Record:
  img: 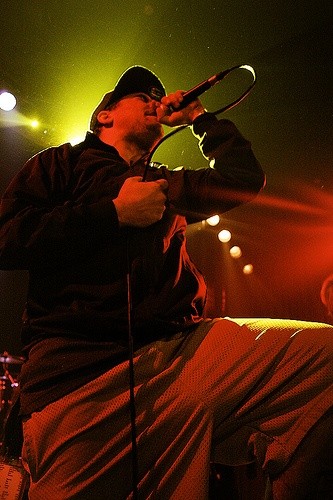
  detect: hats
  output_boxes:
[88,65,167,136]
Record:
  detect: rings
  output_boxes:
[156,180,163,189]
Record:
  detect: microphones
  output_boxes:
[159,65,235,116]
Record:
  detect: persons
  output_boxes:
[2,64,333,500]
